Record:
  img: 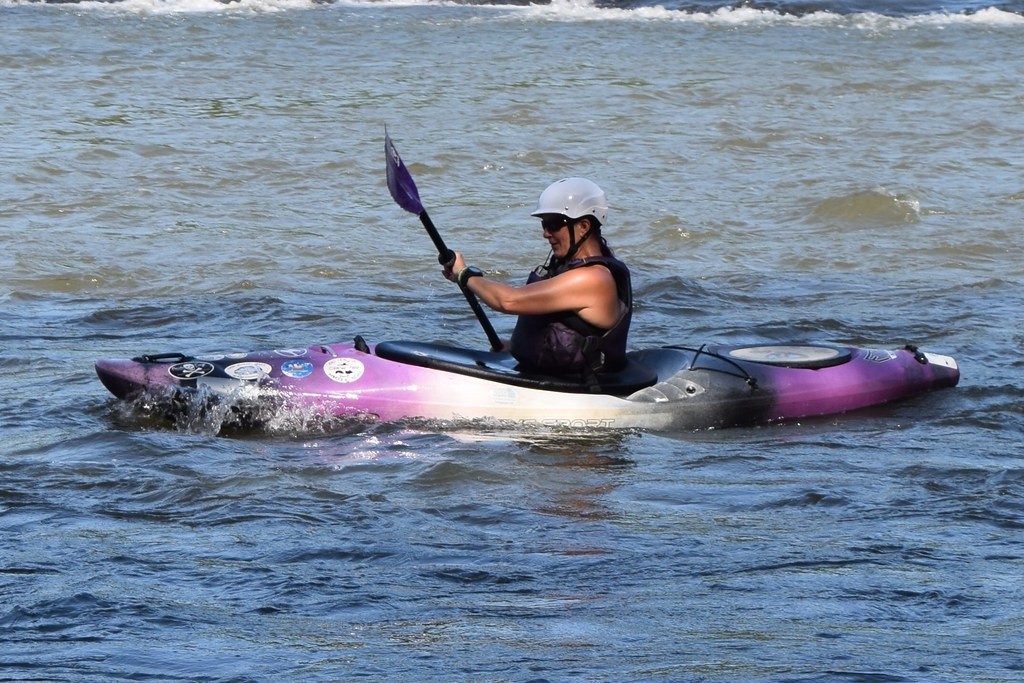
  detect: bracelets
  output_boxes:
[458,266,468,282]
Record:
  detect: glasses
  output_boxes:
[541,218,578,231]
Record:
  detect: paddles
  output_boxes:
[384,125,502,353]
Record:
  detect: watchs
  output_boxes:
[461,266,483,286]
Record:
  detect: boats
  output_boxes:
[95,338,963,440]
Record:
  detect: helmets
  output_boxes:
[531,178,608,225]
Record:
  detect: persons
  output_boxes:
[441,178,631,375]
